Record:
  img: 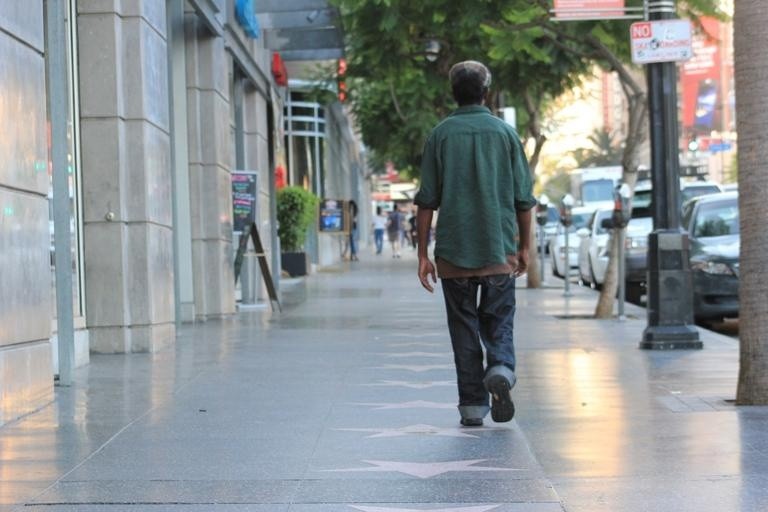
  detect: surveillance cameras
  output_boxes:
[306,9,320,23]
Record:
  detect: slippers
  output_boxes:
[491,375,515,423]
[460,416,484,426]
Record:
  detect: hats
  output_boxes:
[449,60,492,93]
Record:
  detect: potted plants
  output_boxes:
[274,184,321,277]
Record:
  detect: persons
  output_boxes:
[372,203,433,258]
[412,59,538,427]
[349,199,359,262]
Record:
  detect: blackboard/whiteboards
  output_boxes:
[231,170,258,234]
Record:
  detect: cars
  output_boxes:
[537,164,740,330]
